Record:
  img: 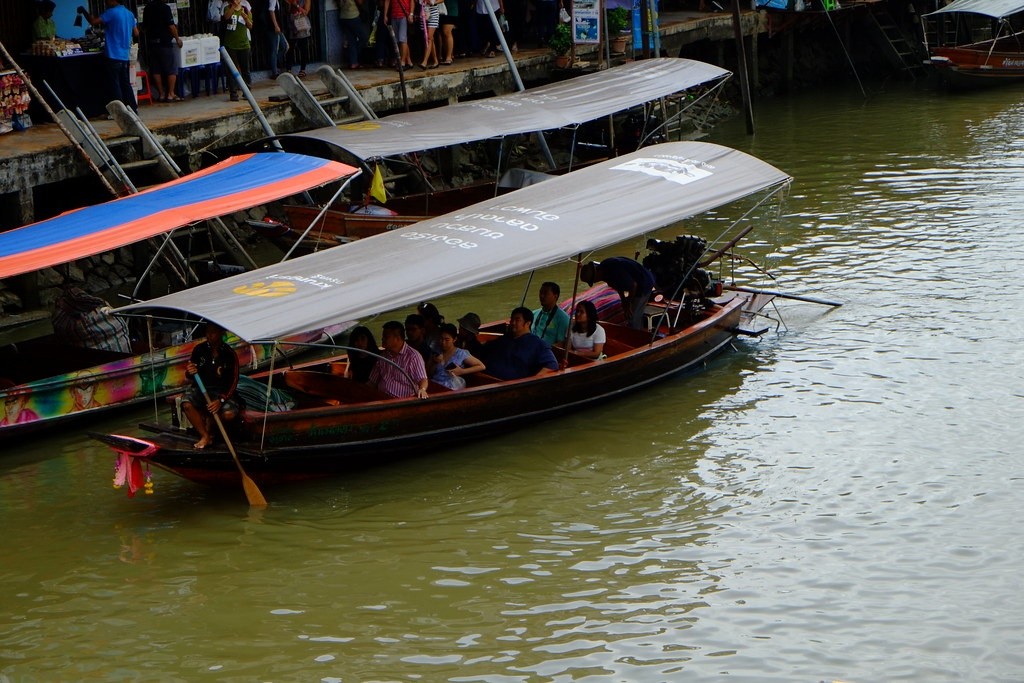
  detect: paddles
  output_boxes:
[720,284,846,306]
[186,357,267,505]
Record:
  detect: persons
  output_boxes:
[418,0,459,69]
[335,0,383,69]
[33,0,55,42]
[405,302,486,390]
[482,307,559,380]
[457,313,486,361]
[471,0,505,58]
[77,0,139,117]
[219,0,253,101]
[181,321,240,449]
[52,289,133,352]
[530,282,569,344]
[368,321,430,399]
[580,256,652,328]
[566,301,606,359]
[383,0,415,71]
[143,0,184,101]
[348,171,394,215]
[344,326,380,381]
[267,0,311,79]
[532,0,564,47]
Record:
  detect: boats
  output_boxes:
[245,57,734,260]
[0,152,361,430]
[920,0,1024,88]
[85,142,795,491]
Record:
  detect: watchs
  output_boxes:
[219,398,225,405]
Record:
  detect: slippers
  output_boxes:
[158,96,167,103]
[167,95,185,102]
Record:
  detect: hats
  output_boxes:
[457,313,481,335]
[581,261,596,288]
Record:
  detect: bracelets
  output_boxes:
[410,13,414,16]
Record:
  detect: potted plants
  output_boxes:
[610,6,628,54]
[548,25,572,69]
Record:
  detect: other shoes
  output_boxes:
[242,88,251,97]
[396,44,518,72]
[298,71,306,78]
[229,94,240,102]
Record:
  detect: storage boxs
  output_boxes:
[172,37,201,70]
[197,36,221,64]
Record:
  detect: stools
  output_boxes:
[176,62,227,100]
[152,324,193,345]
[643,306,670,332]
[136,72,153,108]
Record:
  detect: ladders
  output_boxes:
[56,100,242,287]
[276,65,432,199]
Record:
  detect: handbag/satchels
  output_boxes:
[293,10,312,34]
